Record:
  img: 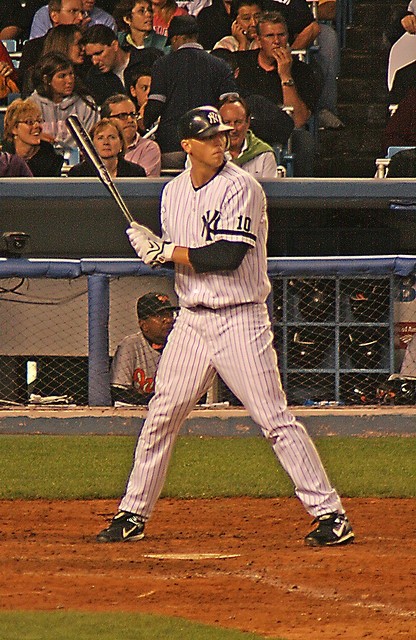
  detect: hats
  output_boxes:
[136,292,181,318]
[163,13,200,48]
[177,105,235,141]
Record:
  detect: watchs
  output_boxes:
[281,77,297,88]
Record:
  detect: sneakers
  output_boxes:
[303,511,356,547]
[316,108,346,130]
[96,511,146,543]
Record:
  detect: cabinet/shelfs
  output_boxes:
[267,276,395,405]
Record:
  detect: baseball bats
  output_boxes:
[65,114,163,271]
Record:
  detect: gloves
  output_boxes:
[138,239,177,267]
[125,220,161,251]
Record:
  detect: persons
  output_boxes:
[196,0,232,50]
[0,40,19,107]
[0,141,35,177]
[78,23,165,106]
[137,14,245,177]
[209,48,295,146]
[183,90,278,179]
[121,0,190,37]
[123,62,154,117]
[1,95,64,176]
[108,290,206,406]
[68,119,146,177]
[93,104,356,549]
[384,2,416,106]
[262,1,347,132]
[210,0,266,52]
[16,0,92,93]
[28,0,119,41]
[99,93,162,176]
[111,0,173,56]
[232,11,319,176]
[20,23,101,100]
[23,49,101,148]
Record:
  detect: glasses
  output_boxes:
[16,119,45,126]
[58,7,89,20]
[108,112,140,121]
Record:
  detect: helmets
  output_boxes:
[348,281,388,322]
[346,334,385,369]
[345,377,376,406]
[379,376,416,405]
[296,281,336,320]
[289,330,331,368]
